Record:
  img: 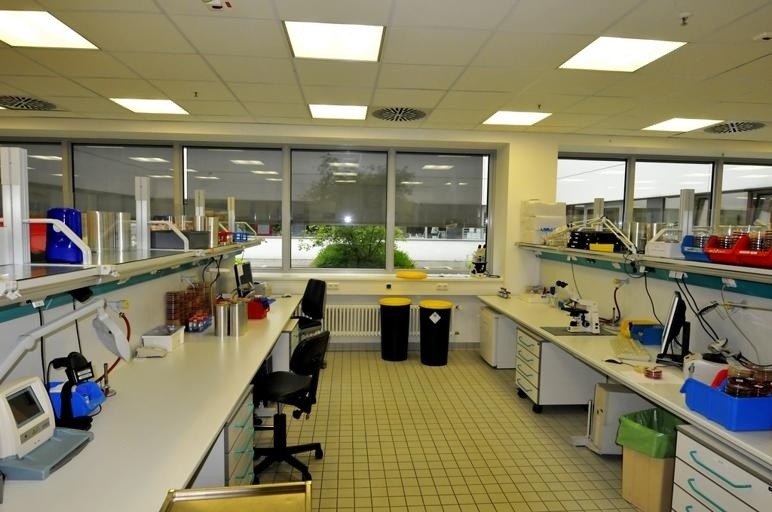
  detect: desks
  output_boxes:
[0,295,304,512]
[477,294,772,511]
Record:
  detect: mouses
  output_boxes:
[281,293,291,298]
[603,357,622,363]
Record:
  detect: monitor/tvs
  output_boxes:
[234,262,255,299]
[660,291,690,360]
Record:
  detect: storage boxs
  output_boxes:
[670,424,771,511]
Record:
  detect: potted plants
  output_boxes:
[470,244,489,274]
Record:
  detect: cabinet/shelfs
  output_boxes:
[515,324,607,414]
[224,385,254,486]
[478,306,516,370]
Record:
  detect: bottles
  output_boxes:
[718,225,771,252]
[663,223,681,244]
[691,226,711,247]
[726,364,771,398]
[189,311,209,332]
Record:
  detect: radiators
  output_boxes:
[291,304,455,337]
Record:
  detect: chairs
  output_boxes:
[289,278,326,341]
[251,330,330,485]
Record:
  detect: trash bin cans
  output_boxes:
[620,407,691,512]
[379,298,452,365]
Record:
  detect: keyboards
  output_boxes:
[608,336,652,361]
